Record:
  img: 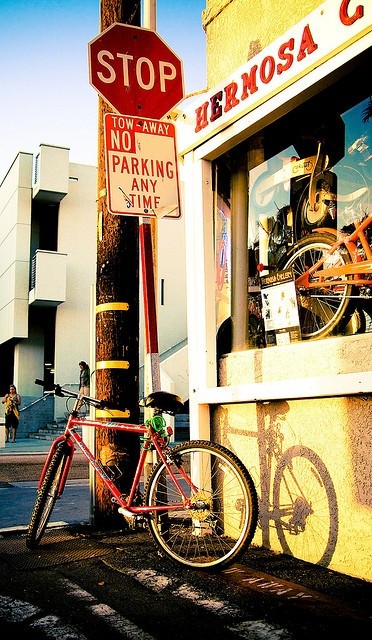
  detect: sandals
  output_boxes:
[5,440,9,443]
[11,440,16,443]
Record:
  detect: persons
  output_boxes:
[75,360,90,412]
[3,383,21,442]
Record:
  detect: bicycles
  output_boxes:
[251,139,334,228]
[24,376,257,572]
[210,397,340,571]
[274,184,372,340]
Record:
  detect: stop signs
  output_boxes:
[86,21,186,120]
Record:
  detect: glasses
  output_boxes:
[10,388,14,390]
